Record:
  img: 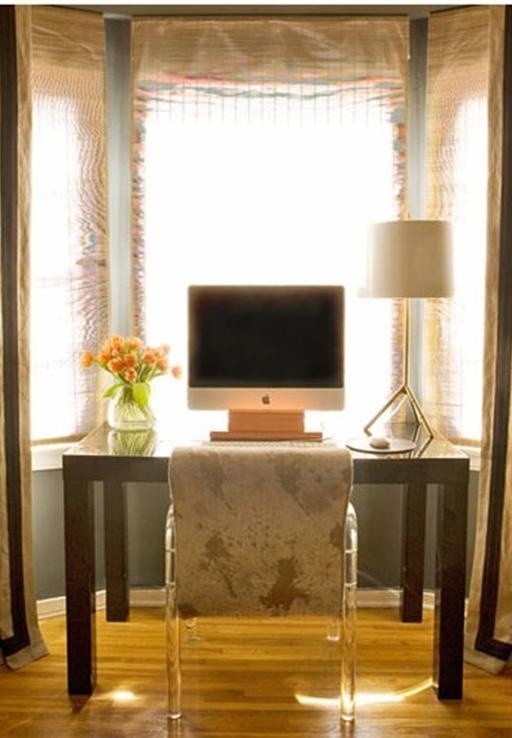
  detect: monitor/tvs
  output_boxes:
[187,284,345,442]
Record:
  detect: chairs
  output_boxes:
[153,444,362,736]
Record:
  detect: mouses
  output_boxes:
[368,434,391,449]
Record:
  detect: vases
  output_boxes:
[107,388,154,437]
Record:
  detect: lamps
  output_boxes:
[361,217,456,456]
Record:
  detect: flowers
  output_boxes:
[80,332,177,429]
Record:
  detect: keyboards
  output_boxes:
[203,441,338,447]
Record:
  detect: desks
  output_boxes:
[60,414,476,692]
